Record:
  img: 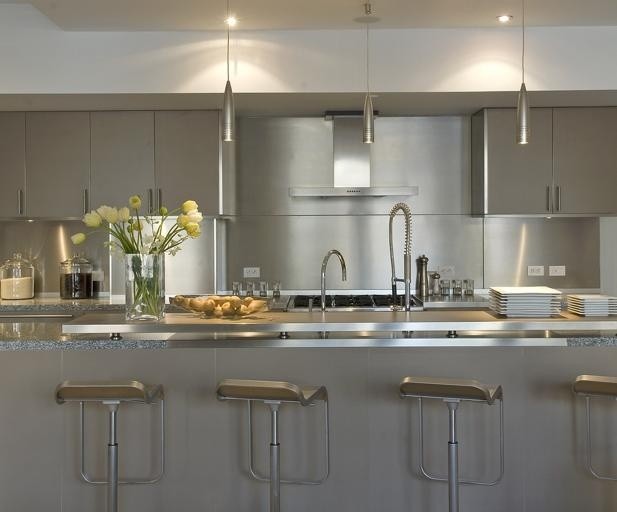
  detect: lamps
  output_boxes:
[516,0,530,145]
[222,0,235,142]
[363,4,374,143]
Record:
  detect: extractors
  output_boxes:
[287,110,419,199]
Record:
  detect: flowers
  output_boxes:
[70,194,205,315]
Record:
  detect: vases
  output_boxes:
[124,253,166,320]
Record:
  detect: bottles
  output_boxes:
[58,251,94,300]
[0,252,36,301]
[417,255,430,297]
[432,270,441,295]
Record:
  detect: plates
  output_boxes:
[486,284,617,319]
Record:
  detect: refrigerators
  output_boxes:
[108,215,227,301]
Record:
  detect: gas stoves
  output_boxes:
[287,291,425,313]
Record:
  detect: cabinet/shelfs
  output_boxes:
[90,109,223,217]
[0,111,91,222]
[470,104,617,220]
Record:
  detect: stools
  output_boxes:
[216,378,330,512]
[398,376,504,512]
[55,380,164,512]
[570,374,617,482]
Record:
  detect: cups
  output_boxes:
[259,280,268,298]
[245,280,255,296]
[464,279,474,296]
[453,279,462,296]
[232,281,242,296]
[271,279,282,298]
[441,280,450,296]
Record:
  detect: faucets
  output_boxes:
[388,203,411,313]
[320,250,347,311]
